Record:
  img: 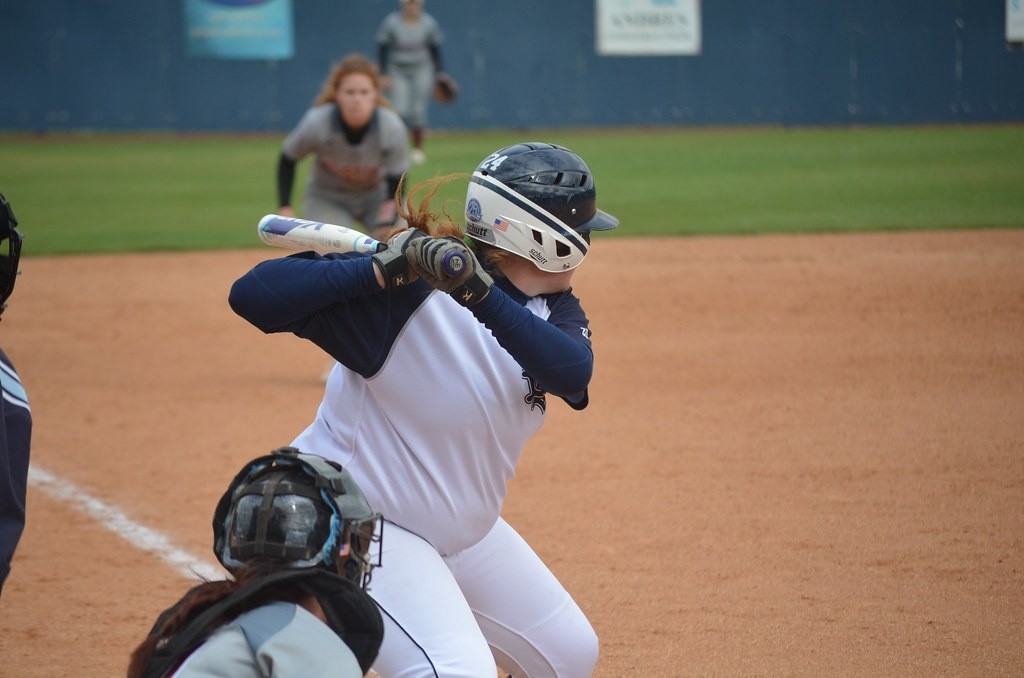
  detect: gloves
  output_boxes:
[407,235,494,308]
[372,226,432,293]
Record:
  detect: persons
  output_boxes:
[127,446,385,678]
[229,141,622,678]
[374,0,457,163]
[0,192,34,598]
[274,53,424,243]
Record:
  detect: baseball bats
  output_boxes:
[256,212,468,279]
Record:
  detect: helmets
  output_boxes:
[212,447,376,576]
[464,141,619,274]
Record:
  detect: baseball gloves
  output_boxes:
[435,72,461,104]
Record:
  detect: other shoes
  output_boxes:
[409,148,423,163]
